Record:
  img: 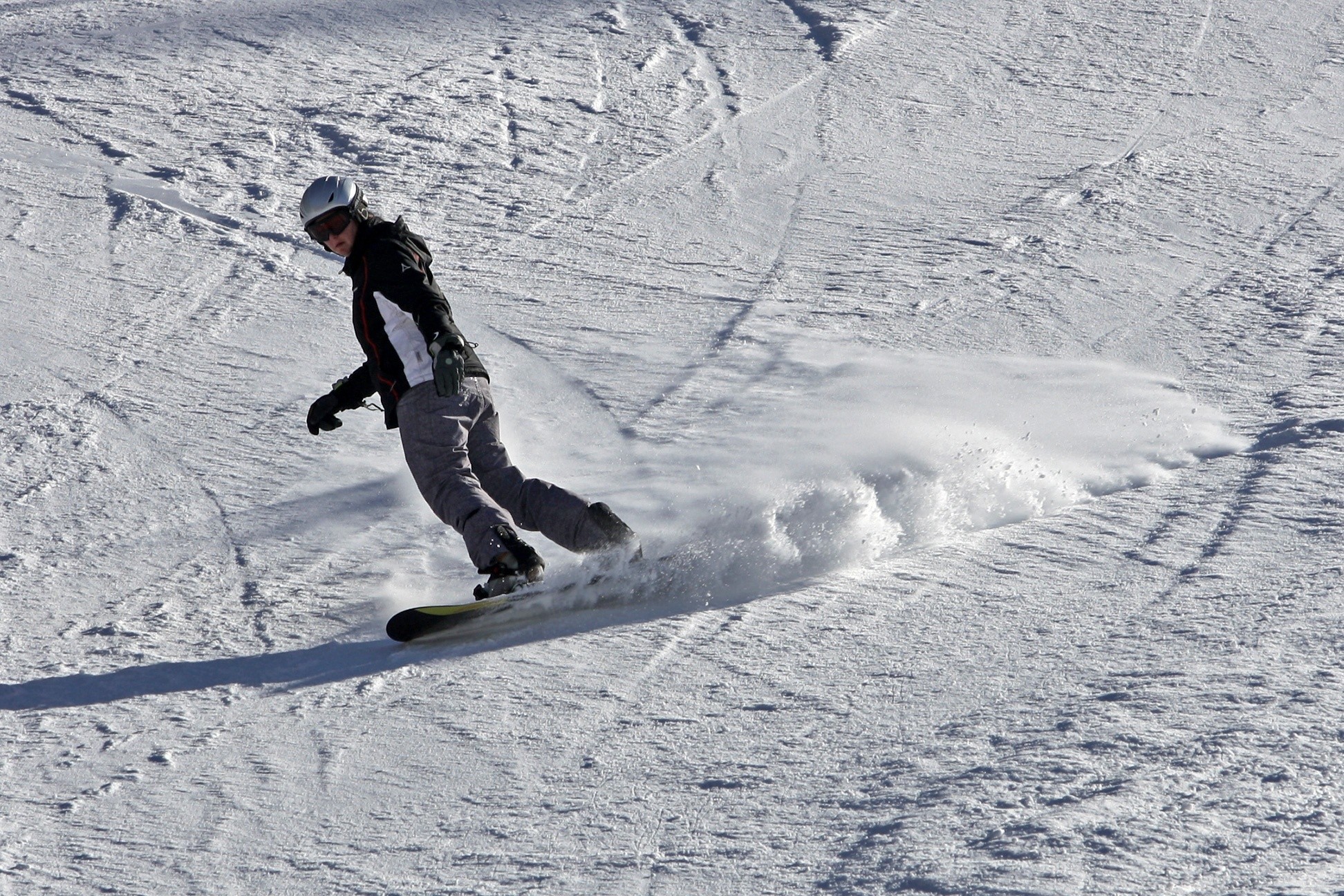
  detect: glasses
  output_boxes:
[306,207,351,242]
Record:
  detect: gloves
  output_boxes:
[429,335,465,397]
[307,390,343,435]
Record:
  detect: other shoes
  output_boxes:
[472,560,547,600]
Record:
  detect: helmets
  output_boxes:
[299,175,368,232]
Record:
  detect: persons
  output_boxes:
[300,175,644,605]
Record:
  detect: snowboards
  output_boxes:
[384,554,681,644]
